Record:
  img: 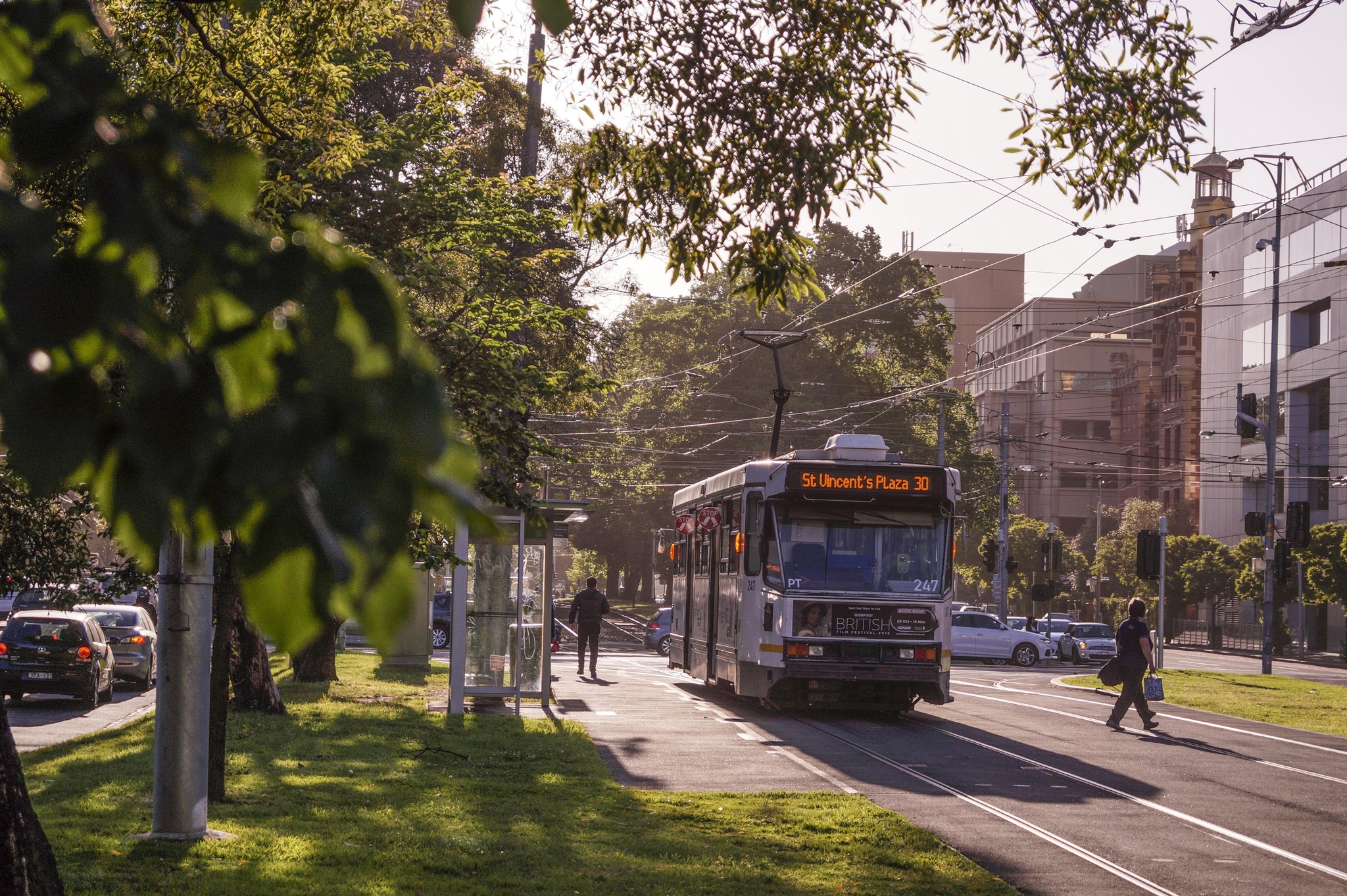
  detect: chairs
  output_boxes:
[785,543,825,580]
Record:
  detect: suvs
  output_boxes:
[950,611,1052,668]
[432,592,562,650]
[1057,622,1117,665]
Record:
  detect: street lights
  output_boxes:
[1226,153,1285,676]
[1199,430,1305,662]
[1019,463,1102,622]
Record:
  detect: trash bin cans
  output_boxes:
[1210,625,1223,649]
[508,623,542,692]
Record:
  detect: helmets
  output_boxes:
[137,586,150,600]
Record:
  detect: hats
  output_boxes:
[800,602,828,617]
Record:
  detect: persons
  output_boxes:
[568,576,610,677]
[555,586,566,599]
[551,603,555,638]
[1106,599,1159,731]
[1026,616,1039,634]
[797,600,828,638]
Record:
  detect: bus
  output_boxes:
[658,328,970,716]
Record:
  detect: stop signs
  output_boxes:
[732,506,740,530]
[675,514,696,535]
[699,506,722,530]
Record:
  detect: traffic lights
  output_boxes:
[1050,580,1062,598]
[1006,556,1019,574]
[553,573,557,578]
[1031,583,1049,602]
[982,538,995,573]
[1274,545,1291,579]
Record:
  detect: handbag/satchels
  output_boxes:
[550,638,560,653]
[1097,656,1122,686]
[1144,671,1164,701]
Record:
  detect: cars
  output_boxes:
[0,609,121,709]
[951,601,1074,655]
[644,607,671,657]
[54,603,158,690]
[0,568,160,636]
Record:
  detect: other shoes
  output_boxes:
[1143,721,1159,730]
[576,670,584,675]
[591,672,597,677]
[1106,718,1125,731]
